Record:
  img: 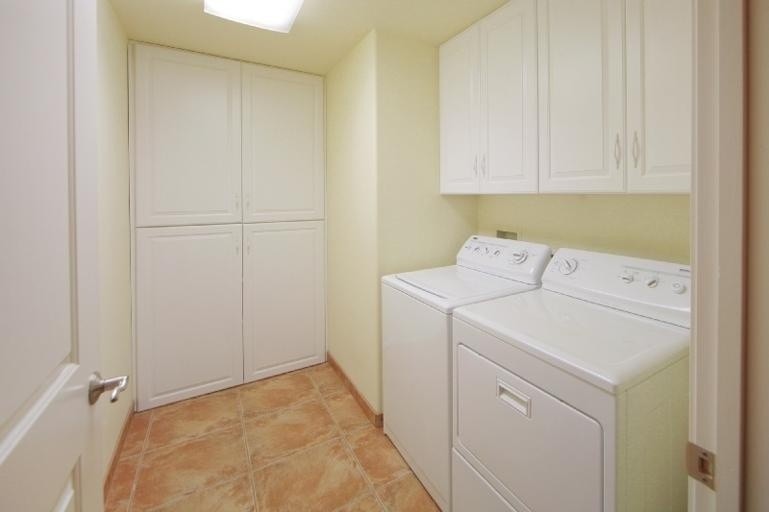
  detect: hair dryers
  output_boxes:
[451,248,692,512]
[381,235,552,512]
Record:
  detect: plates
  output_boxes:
[437,1,538,198]
[538,0,695,197]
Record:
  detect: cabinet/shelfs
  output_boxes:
[538,0,695,197]
[130,221,329,411]
[437,1,538,198]
[126,42,331,227]
